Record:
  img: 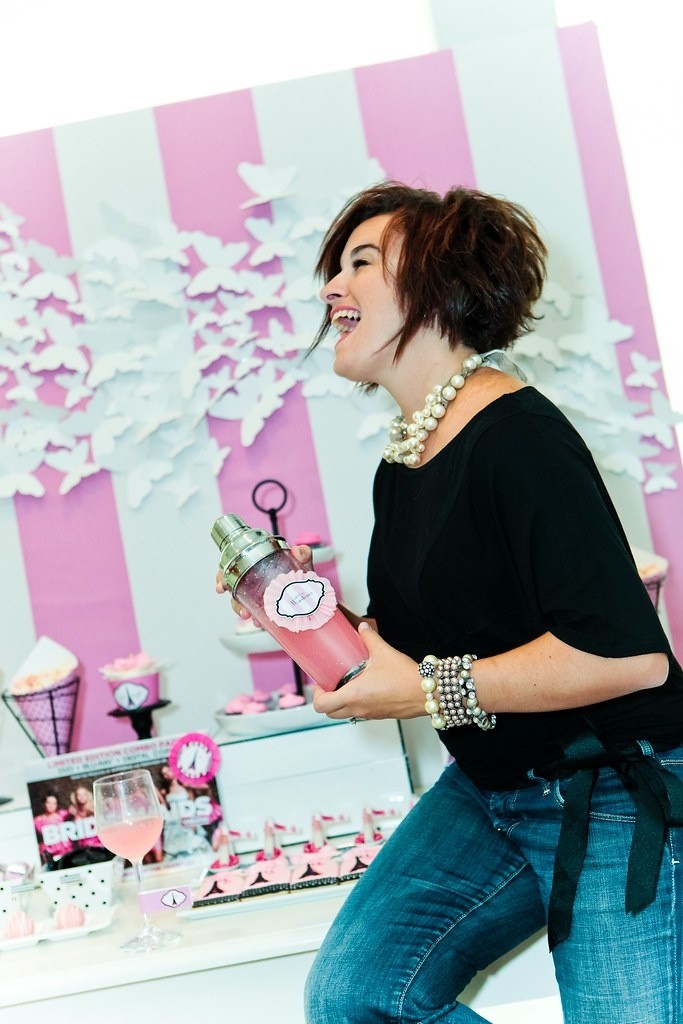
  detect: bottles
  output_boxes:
[210,514,371,694]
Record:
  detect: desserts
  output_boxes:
[295,532,321,548]
[224,681,305,714]
[235,616,262,634]
[197,832,384,905]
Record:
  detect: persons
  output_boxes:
[35,764,222,871]
[214,179,683,1024]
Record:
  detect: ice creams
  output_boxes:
[97,652,158,676]
[9,635,81,759]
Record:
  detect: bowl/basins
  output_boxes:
[0,857,129,911]
[104,668,160,710]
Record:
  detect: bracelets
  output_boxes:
[417,653,497,732]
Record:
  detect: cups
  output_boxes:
[134,884,192,915]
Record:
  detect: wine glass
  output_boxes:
[92,769,184,955]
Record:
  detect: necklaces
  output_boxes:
[382,349,528,468]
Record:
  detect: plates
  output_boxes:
[214,685,324,736]
[0,905,119,951]
[219,630,285,654]
[179,858,362,923]
[203,793,419,864]
